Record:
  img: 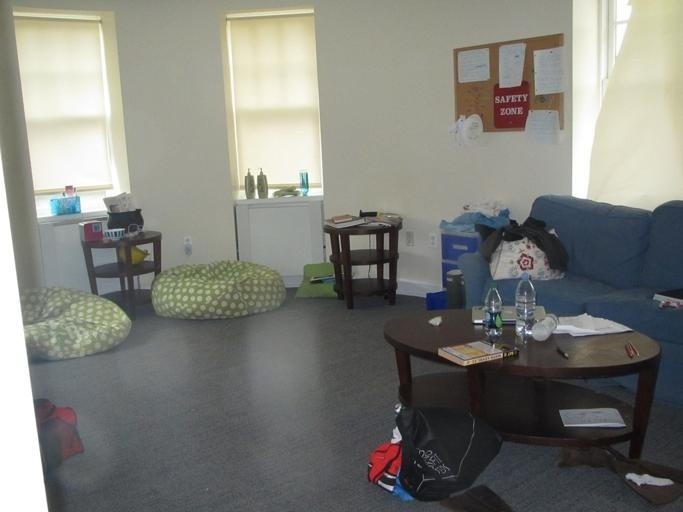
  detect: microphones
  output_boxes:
[19,286,132,363]
[150,260,285,321]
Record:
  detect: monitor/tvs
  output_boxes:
[558,407,627,430]
[332,216,353,223]
[324,215,360,230]
[437,338,518,367]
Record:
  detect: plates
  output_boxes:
[441,232,482,291]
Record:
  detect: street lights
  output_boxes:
[398,405,502,501]
[489,228,565,280]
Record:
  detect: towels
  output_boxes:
[447,269,466,308]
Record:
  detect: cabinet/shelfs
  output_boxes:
[299,170,309,197]
[484,273,559,343]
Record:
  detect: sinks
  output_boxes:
[456,193,682,408]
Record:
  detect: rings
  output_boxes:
[557,347,569,359]
[625,341,639,359]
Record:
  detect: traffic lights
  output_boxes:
[295,263,337,297]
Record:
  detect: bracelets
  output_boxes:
[103,228,126,241]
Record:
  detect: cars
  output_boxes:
[128,224,144,238]
